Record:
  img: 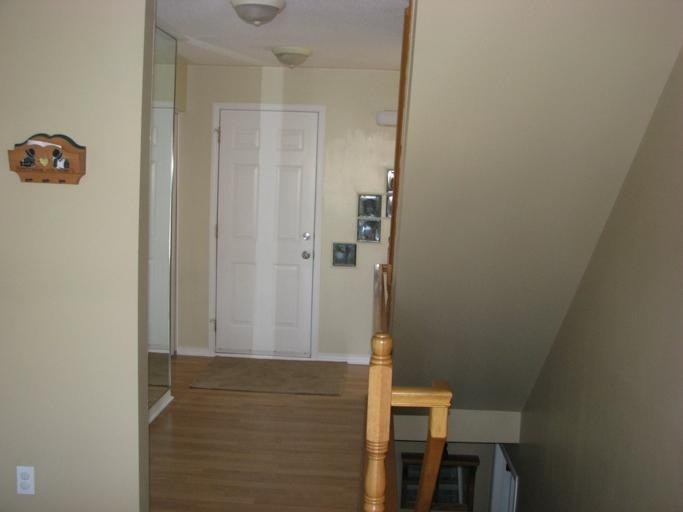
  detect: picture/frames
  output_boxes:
[331,168,394,268]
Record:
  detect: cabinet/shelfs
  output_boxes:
[402,454,480,512]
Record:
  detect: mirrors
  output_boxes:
[147,27,176,423]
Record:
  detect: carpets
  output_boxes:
[188,356,348,397]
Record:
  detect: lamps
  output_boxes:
[231,0,313,71]
